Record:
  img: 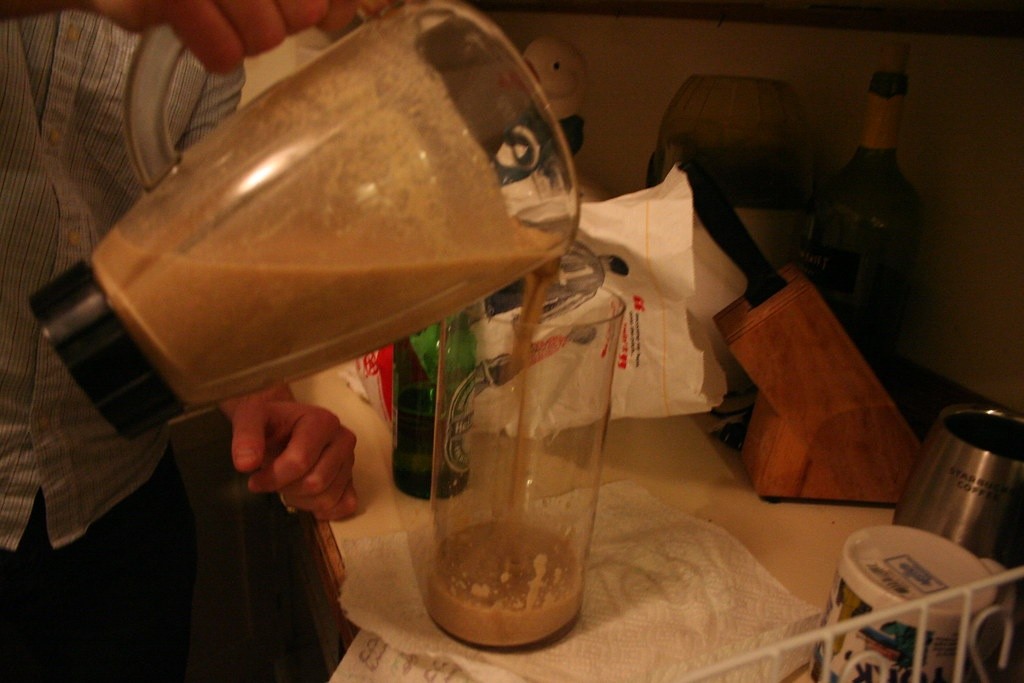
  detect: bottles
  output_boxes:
[801,41,924,390]
[392,302,478,499]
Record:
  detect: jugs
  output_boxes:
[28,0,582,441]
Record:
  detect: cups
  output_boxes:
[891,401,1024,570]
[805,524,1015,683]
[418,287,628,654]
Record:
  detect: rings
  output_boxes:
[280,492,296,515]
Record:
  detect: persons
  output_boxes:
[0,0,384,683]
